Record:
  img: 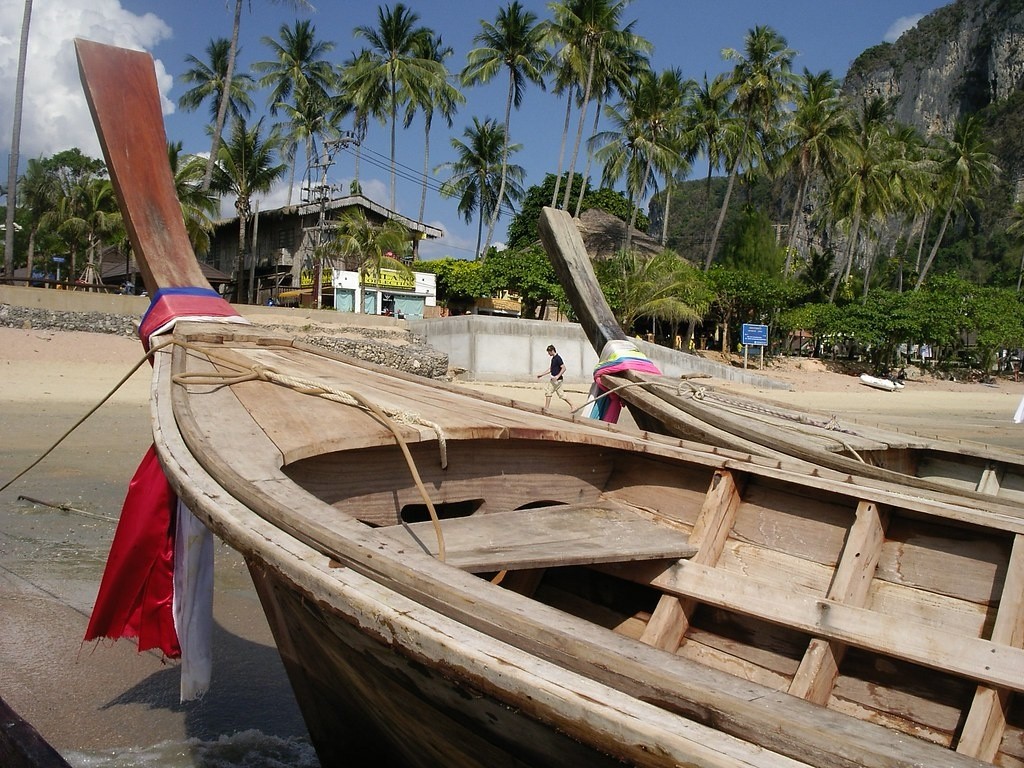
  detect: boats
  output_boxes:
[70,37,1023,762]
[861,373,906,393]
[539,204,1024,510]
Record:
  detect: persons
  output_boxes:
[383,307,404,319]
[897,368,906,379]
[537,345,576,413]
[806,344,814,357]
[1014,363,1020,382]
